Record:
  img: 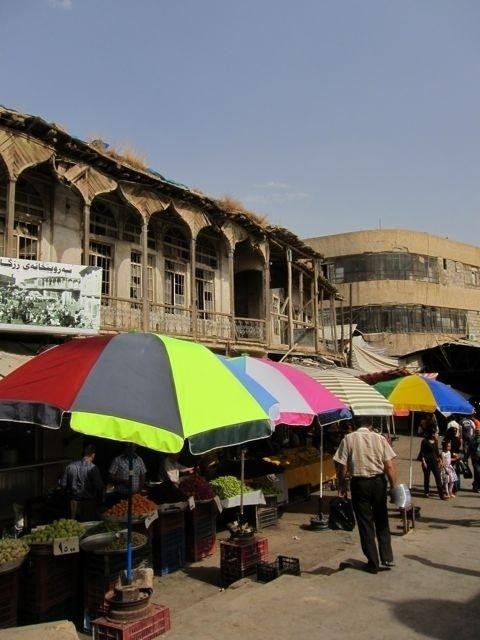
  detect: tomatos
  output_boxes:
[178,474,215,499]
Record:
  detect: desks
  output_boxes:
[214,447,338,531]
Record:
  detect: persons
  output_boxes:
[58,445,104,521]
[152,452,192,484]
[334,416,397,573]
[109,442,147,501]
[198,447,224,481]
[289,430,300,446]
[417,411,480,500]
[257,437,311,478]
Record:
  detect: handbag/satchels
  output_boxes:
[328,495,355,532]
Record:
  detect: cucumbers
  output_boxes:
[145,480,187,505]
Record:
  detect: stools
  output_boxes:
[387,502,416,534]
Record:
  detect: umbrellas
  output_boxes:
[292,362,394,499]
[223,351,352,518]
[371,372,474,490]
[0,327,278,583]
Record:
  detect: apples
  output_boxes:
[0,518,86,565]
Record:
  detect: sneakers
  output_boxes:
[423,487,480,500]
[363,560,396,574]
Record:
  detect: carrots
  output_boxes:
[102,493,157,517]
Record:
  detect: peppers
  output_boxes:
[210,475,249,500]
[82,518,121,550]
